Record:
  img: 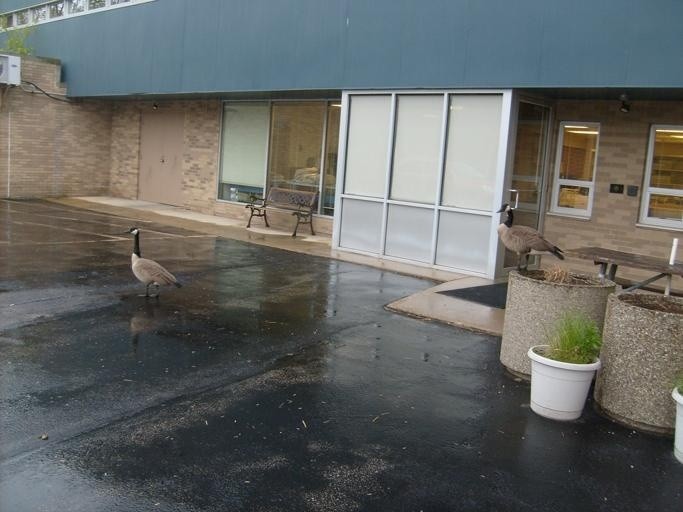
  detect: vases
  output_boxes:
[497,263,682,440]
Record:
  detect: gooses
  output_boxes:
[124,227,182,297]
[496,204,565,272]
[130,303,179,353]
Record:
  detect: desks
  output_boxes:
[559,243,682,296]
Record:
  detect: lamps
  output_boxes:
[616,92,633,114]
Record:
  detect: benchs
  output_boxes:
[242,184,320,239]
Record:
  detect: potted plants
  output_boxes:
[669,366,683,465]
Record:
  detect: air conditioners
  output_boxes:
[0,54,21,87]
